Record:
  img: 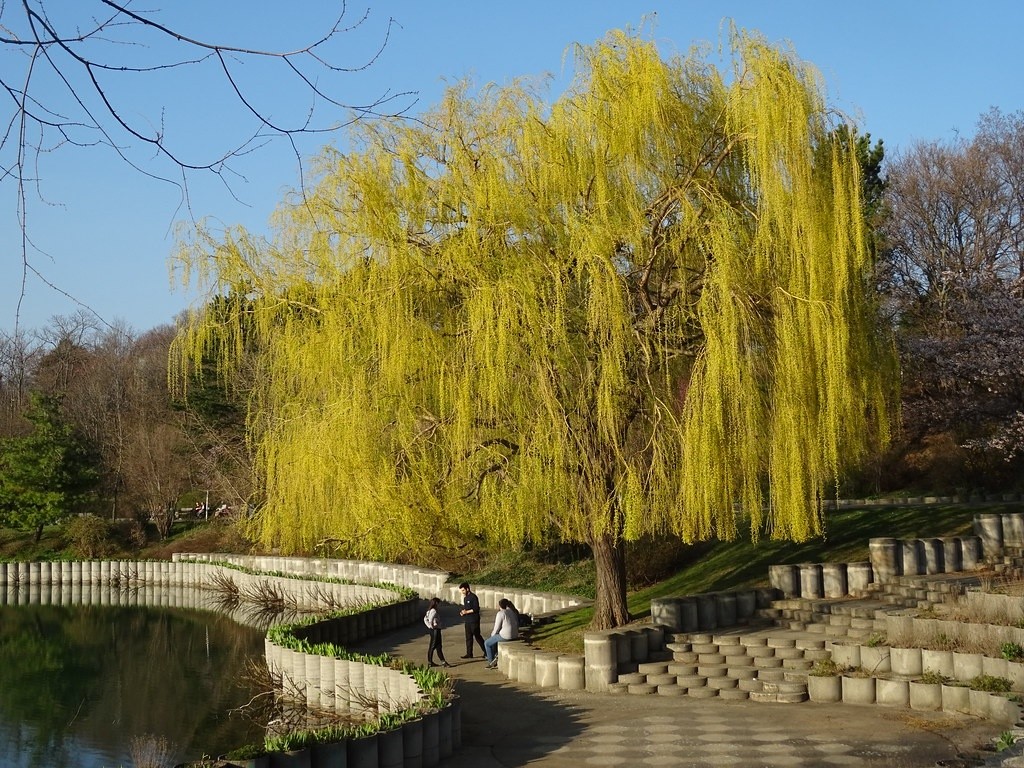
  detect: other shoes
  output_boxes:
[460,654,474,658]
[489,655,499,667]
[484,666,494,670]
[441,662,451,668]
[428,662,440,667]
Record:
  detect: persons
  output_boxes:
[193,502,206,519]
[424,598,452,668]
[485,599,520,670]
[459,582,488,659]
